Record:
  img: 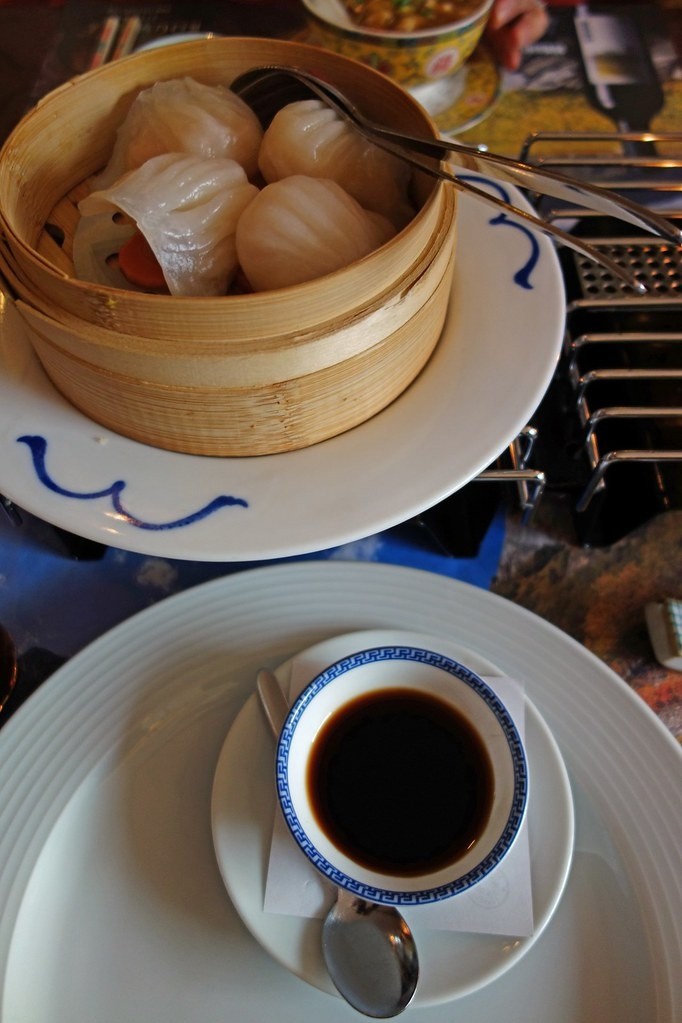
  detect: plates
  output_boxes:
[0,565,682,1022]
[132,32,219,54]
[212,631,575,1009]
[293,27,503,136]
[0,135,566,561]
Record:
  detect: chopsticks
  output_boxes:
[90,14,141,75]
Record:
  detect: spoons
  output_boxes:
[228,66,682,294]
[256,669,419,1018]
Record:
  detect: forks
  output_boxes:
[236,70,649,317]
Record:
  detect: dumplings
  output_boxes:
[77,75,416,298]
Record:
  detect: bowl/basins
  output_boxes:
[304,1,492,89]
[277,646,528,905]
